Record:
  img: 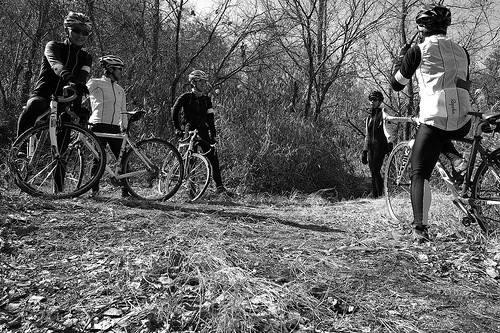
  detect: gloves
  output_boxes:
[399,44,410,56]
[64,75,73,83]
[75,107,88,120]
[362,151,366,164]
[175,129,182,136]
[212,137,219,145]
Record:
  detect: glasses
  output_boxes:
[109,65,123,71]
[369,99,380,102]
[67,28,90,36]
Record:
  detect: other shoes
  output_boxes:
[413,226,429,242]
[217,189,235,197]
[92,191,102,201]
[451,159,468,181]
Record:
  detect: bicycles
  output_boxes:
[382,107,500,242]
[158,129,217,204]
[7,80,184,200]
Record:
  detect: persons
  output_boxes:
[15,11,94,193]
[362,91,393,198]
[81,54,130,197]
[172,70,234,197]
[391,3,473,244]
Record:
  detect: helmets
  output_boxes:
[415,6,451,27]
[63,12,92,33]
[188,70,209,83]
[99,55,124,68]
[369,91,383,102]
